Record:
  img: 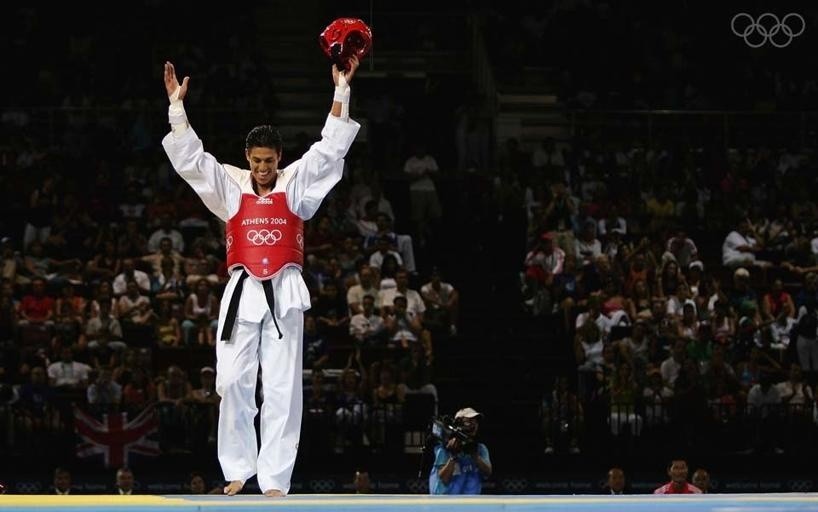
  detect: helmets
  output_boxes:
[318,17,372,72]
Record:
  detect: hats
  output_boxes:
[455,407,484,419]
